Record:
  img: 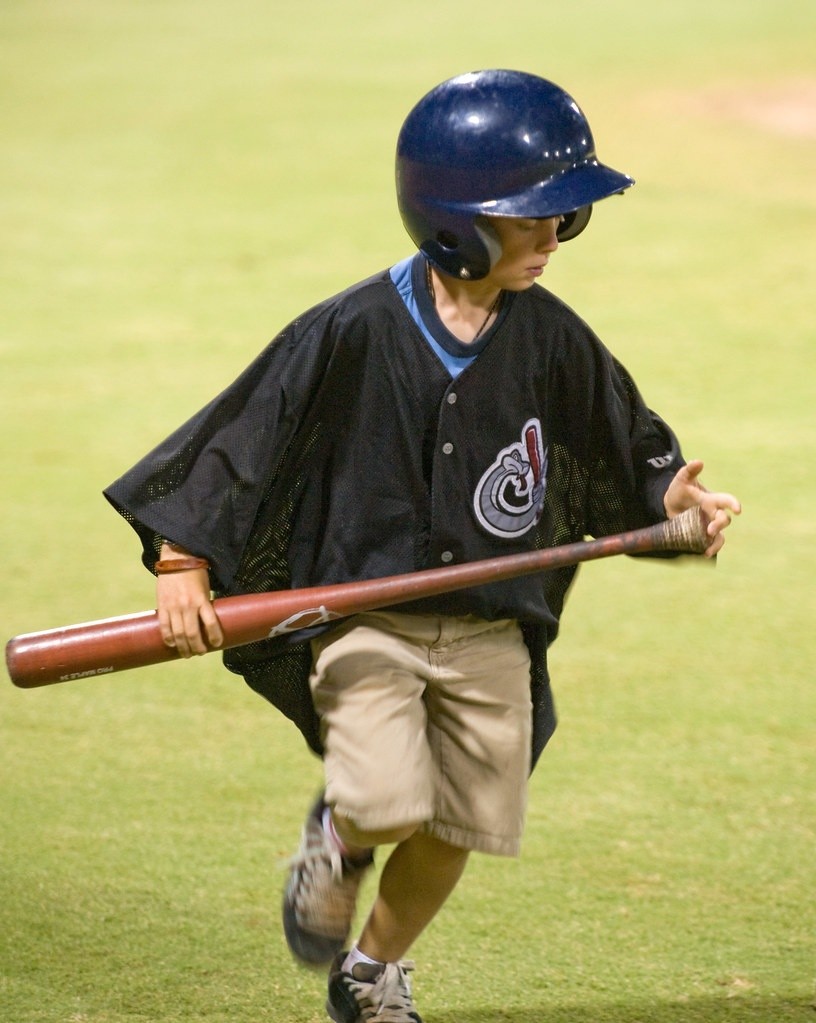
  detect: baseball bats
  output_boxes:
[5,501,713,689]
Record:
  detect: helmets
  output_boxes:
[393,67,634,280]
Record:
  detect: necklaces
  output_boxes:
[420,258,503,344]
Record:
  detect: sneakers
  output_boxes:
[325,950,423,1023]
[280,793,377,965]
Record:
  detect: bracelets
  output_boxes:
[154,556,210,575]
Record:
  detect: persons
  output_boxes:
[103,69,741,1023]
